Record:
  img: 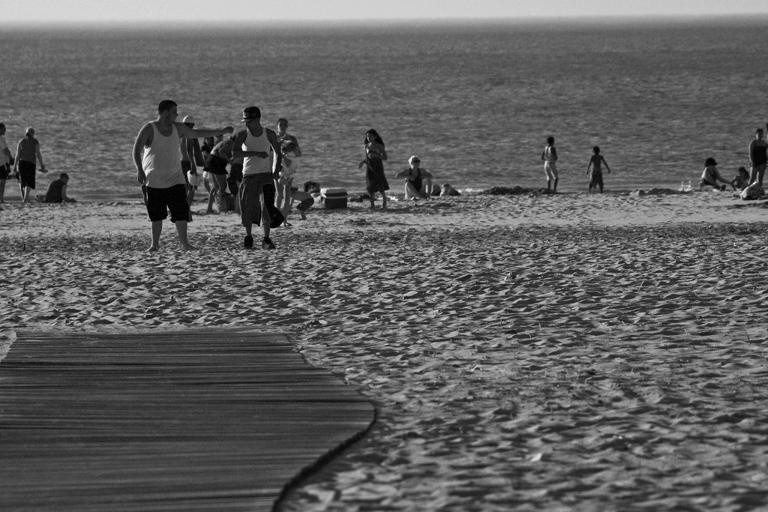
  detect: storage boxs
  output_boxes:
[321,188,349,207]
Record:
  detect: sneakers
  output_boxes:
[262,237,275,249]
[244,236,253,248]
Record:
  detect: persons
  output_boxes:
[699,130,768,200]
[394,156,434,200]
[132,99,314,254]
[0,121,77,205]
[541,137,559,191]
[358,128,390,209]
[586,146,611,193]
